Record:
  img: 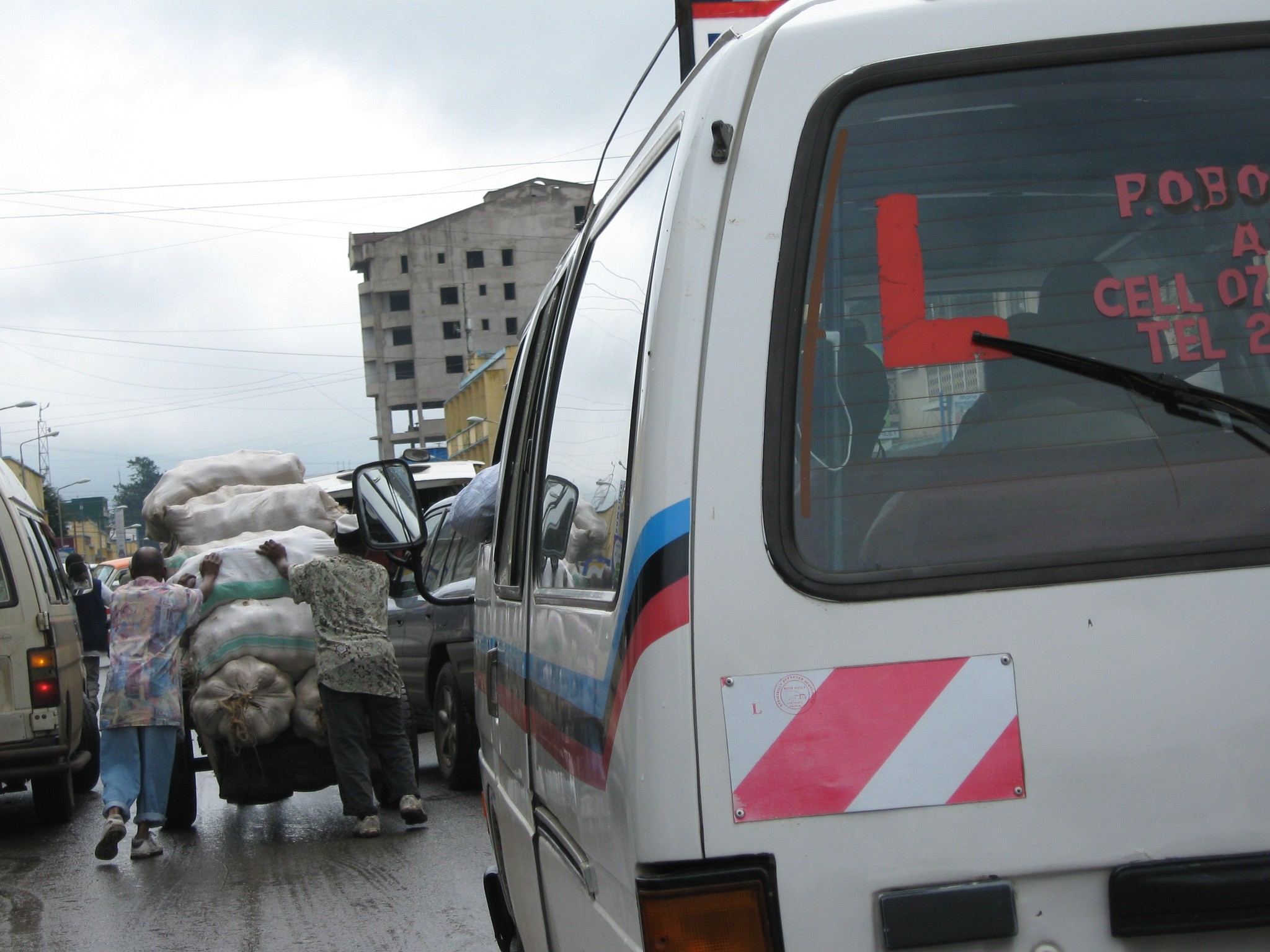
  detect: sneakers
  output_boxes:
[400,793,428,825]
[94,814,126,860]
[352,815,381,836]
[128,829,163,859]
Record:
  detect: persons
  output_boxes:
[933,261,1223,459]
[256,512,427,837]
[809,337,890,466]
[95,546,223,860]
[69,570,90,589]
[66,554,114,714]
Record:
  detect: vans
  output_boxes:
[349,0,1270,952]
[0,457,103,823]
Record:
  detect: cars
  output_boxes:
[84,555,133,626]
[300,461,484,792]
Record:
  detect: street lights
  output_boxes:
[0,400,36,459]
[20,431,60,489]
[98,504,130,567]
[57,479,91,549]
[105,523,141,560]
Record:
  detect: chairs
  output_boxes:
[798,311,1201,436]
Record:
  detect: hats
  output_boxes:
[335,513,361,539]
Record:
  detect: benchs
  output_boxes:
[792,426,1270,575]
